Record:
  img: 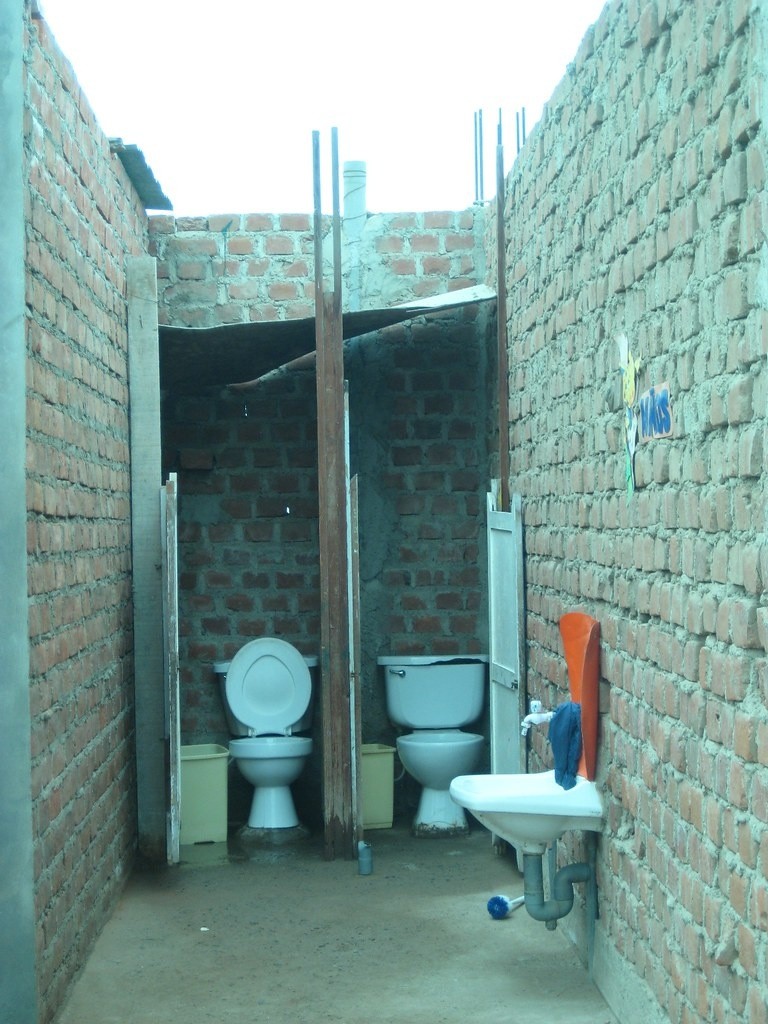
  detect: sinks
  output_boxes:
[451,769,605,842]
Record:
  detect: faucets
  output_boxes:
[521,700,559,736]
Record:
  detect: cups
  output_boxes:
[359,841,374,876]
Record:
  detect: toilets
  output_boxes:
[377,655,489,841]
[213,636,321,829]
